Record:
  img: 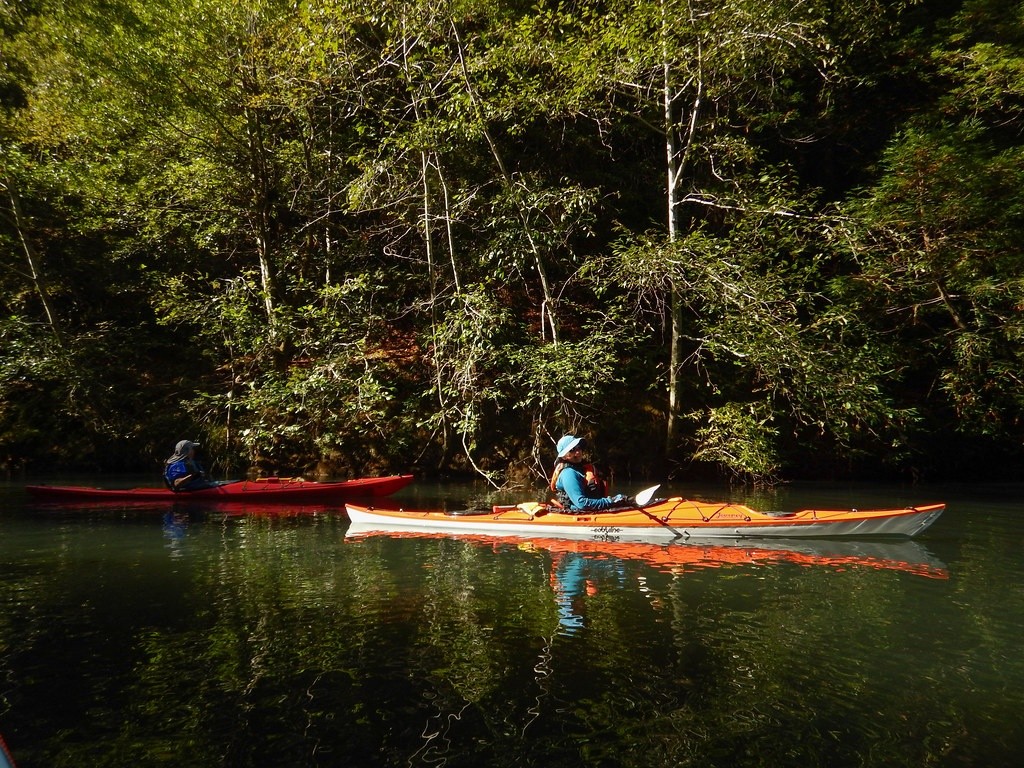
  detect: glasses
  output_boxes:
[569,446,582,453]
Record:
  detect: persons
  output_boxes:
[165,440,217,491]
[551,435,627,512]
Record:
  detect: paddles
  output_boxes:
[620,481,665,509]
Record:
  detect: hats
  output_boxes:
[556,435,587,457]
[166,439,200,464]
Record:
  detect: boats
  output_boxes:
[0,466,415,501]
[344,496,946,537]
